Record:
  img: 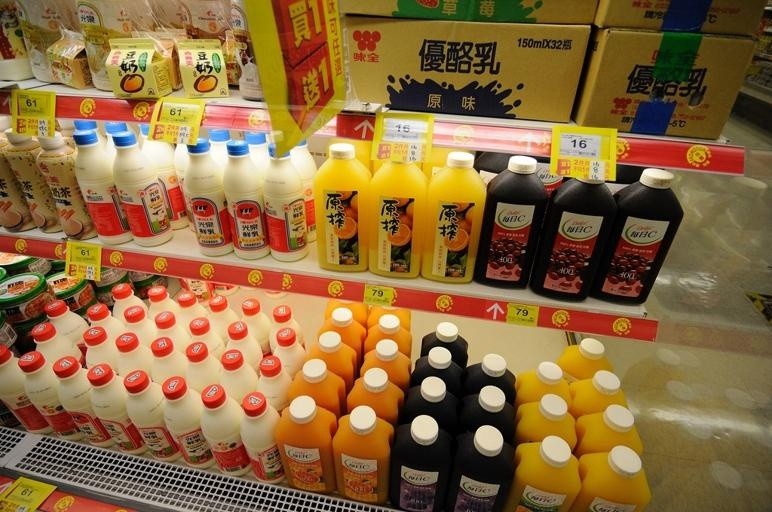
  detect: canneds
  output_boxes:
[0,252,169,352]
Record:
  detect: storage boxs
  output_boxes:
[572,27,762,141]
[593,1,766,37]
[346,16,592,123]
[338,0,599,25]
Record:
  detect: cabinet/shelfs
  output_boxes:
[741,0,772,104]
[0,79,746,512]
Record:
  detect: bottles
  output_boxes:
[104,121,128,158]
[264,144,308,262]
[35,130,96,241]
[205,130,233,177]
[241,298,272,358]
[200,384,251,478]
[572,372,633,415]
[137,124,189,230]
[41,299,87,343]
[331,405,392,503]
[311,144,372,273]
[0,115,13,140]
[517,394,578,449]
[123,306,158,343]
[424,147,468,183]
[2,130,64,234]
[221,350,258,407]
[162,377,216,469]
[461,384,517,436]
[189,318,226,362]
[111,132,173,247]
[151,338,184,385]
[325,298,368,321]
[0,135,37,233]
[116,334,151,375]
[184,140,233,257]
[147,286,183,317]
[393,415,450,511]
[467,354,514,396]
[605,162,662,196]
[411,346,465,397]
[112,283,147,321]
[275,395,337,495]
[560,338,612,379]
[273,328,304,374]
[397,377,460,435]
[239,392,283,483]
[420,151,486,285]
[285,360,346,421]
[74,120,107,162]
[359,340,413,393]
[506,436,582,512]
[123,370,184,463]
[450,424,513,512]
[175,126,198,235]
[57,118,79,154]
[222,141,270,260]
[18,351,82,443]
[345,367,403,427]
[244,131,267,187]
[203,294,237,344]
[473,156,546,290]
[572,445,652,512]
[363,313,411,357]
[474,151,516,187]
[0,347,53,434]
[225,320,263,376]
[86,302,124,347]
[313,307,365,366]
[82,328,118,376]
[53,357,115,448]
[87,364,147,454]
[268,304,307,354]
[31,323,85,364]
[185,342,222,394]
[522,156,568,196]
[73,130,131,243]
[325,138,379,178]
[281,137,317,242]
[178,294,206,327]
[306,332,357,394]
[587,168,682,307]
[529,161,615,302]
[577,404,644,455]
[516,363,574,409]
[370,303,411,330]
[368,148,427,278]
[154,313,192,353]
[422,321,470,362]
[371,140,427,179]
[258,354,294,413]
